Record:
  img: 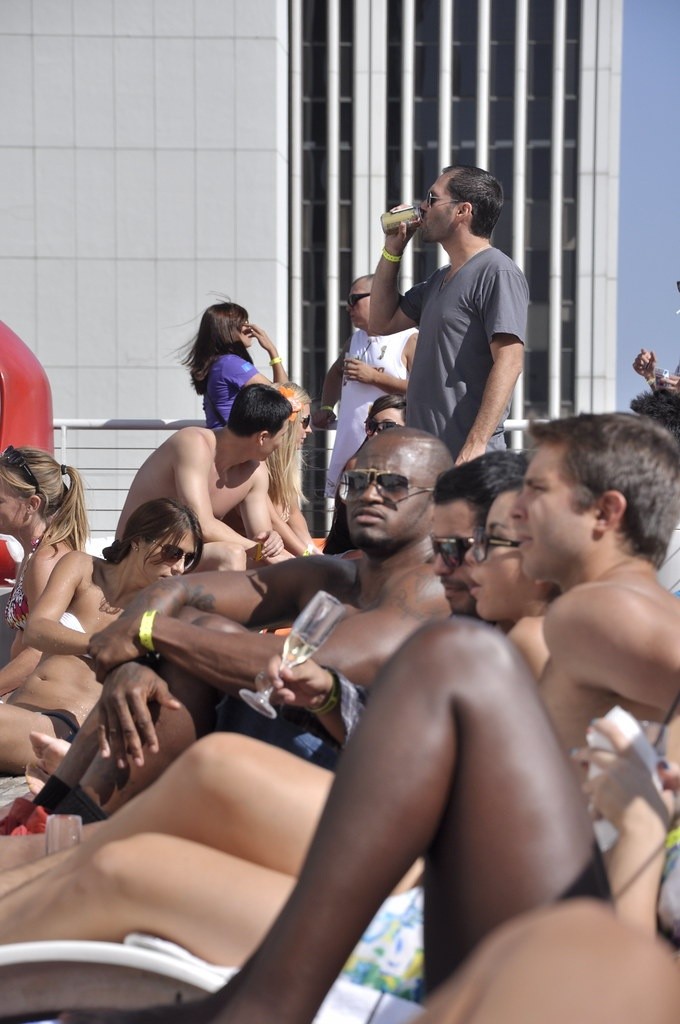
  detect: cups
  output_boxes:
[45,813,82,855]
[345,353,358,379]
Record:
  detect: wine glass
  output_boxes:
[238,589,346,720]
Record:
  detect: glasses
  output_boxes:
[336,467,443,502]
[469,525,521,564]
[365,417,406,440]
[142,534,197,568]
[345,292,371,309]
[428,536,475,569]
[425,194,478,218]
[298,414,313,431]
[2,445,40,496]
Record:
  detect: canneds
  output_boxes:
[380,207,423,235]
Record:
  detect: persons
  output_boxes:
[1,382,680,1024]
[181,303,290,431]
[312,275,420,559]
[368,164,531,472]
[632,280,680,394]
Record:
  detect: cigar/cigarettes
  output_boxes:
[676,309,679,315]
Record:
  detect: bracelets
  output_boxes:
[139,608,158,652]
[269,357,281,366]
[256,540,265,562]
[320,406,334,410]
[382,246,403,261]
[303,683,341,714]
[647,379,655,386]
[302,549,310,557]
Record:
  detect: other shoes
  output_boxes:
[0,797,35,835]
[9,805,50,837]
